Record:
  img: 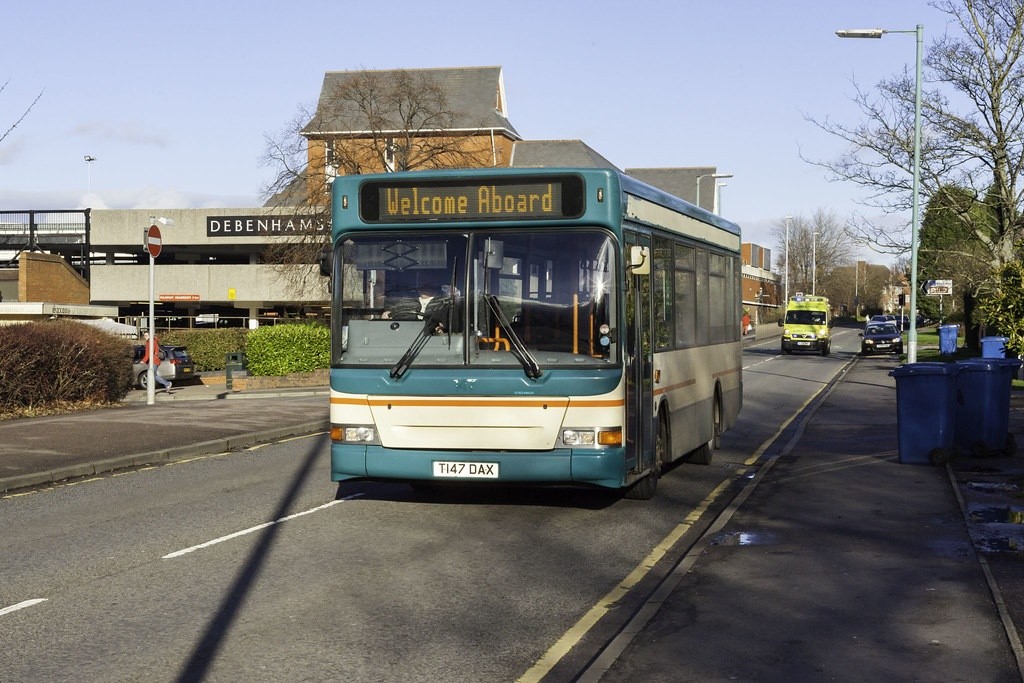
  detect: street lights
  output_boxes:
[813,232,819,296]
[84,156,95,208]
[696,173,734,207]
[785,216,792,304]
[835,25,923,363]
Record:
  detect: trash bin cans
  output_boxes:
[958,356,1023,468]
[935,321,960,356]
[887,360,971,464]
[981,335,1007,359]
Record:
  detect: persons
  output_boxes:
[793,314,798,320]
[812,315,820,322]
[141,331,172,396]
[743,314,750,335]
[381,270,458,335]
[865,314,870,325]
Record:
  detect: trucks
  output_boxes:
[778,296,831,356]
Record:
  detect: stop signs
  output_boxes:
[146,225,162,257]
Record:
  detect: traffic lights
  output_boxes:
[899,294,903,305]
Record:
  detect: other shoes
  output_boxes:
[165,382,172,393]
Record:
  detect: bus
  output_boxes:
[316,168,743,500]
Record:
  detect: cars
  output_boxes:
[858,323,904,356]
[132,345,195,389]
[864,315,910,327]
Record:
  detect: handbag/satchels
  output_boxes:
[157,347,166,360]
